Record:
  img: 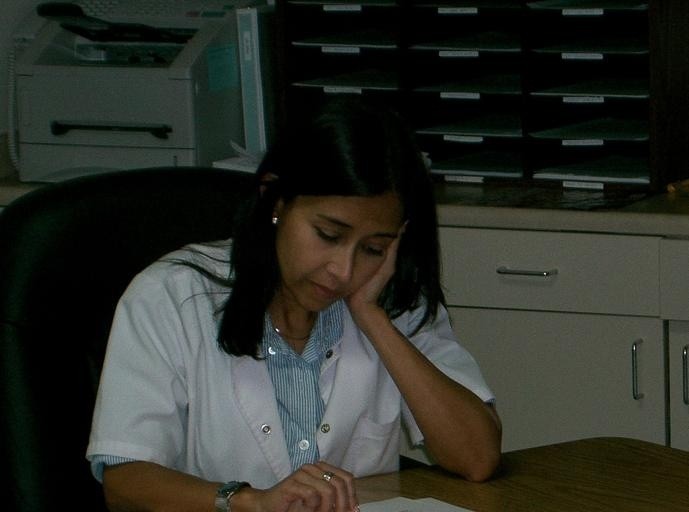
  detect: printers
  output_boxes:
[6,0,246,184]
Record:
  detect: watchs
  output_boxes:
[214,481,250,512]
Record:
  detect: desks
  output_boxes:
[339,435,688,512]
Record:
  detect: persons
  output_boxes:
[84,110,503,512]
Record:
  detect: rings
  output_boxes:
[322,471,335,483]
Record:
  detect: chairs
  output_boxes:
[1,168,426,511]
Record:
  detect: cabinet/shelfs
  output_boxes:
[398,216,687,465]
[268,0,665,199]
